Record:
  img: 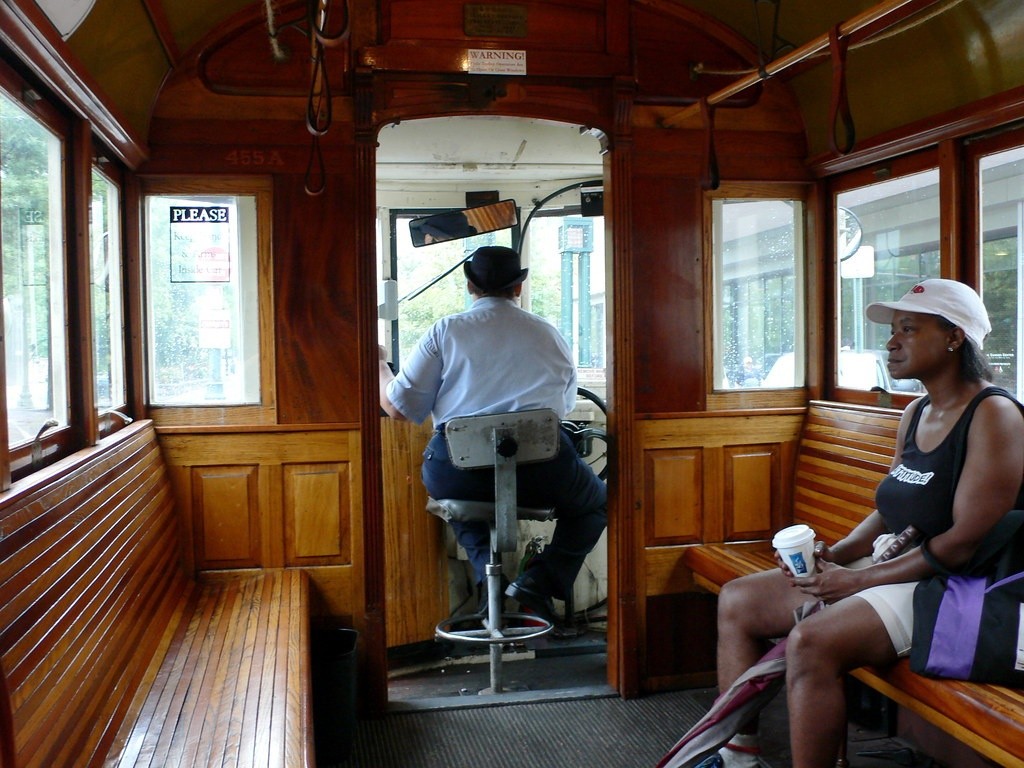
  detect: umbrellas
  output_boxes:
[653,523,917,768]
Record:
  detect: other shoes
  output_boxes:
[693,754,760,768]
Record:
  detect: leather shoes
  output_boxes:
[504,574,553,612]
[478,575,513,615]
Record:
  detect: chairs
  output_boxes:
[424,406,562,691]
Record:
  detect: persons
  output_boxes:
[419,211,477,245]
[377,245,608,632]
[692,276,1024,768]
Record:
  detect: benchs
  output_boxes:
[680,400,1024,767]
[0,419,317,768]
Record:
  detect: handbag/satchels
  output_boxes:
[909,536,1024,687]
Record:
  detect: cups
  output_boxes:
[772,525,817,576]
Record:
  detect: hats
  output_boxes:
[464,245,527,293]
[419,211,477,239]
[866,279,992,351]
